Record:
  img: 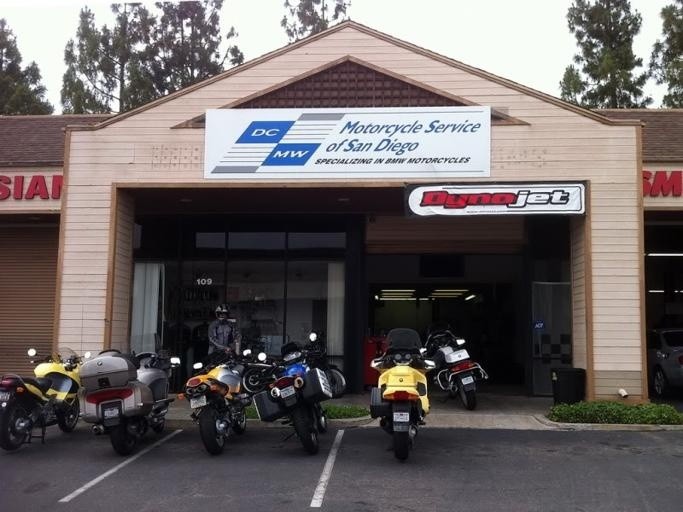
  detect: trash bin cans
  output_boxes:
[551,368,586,404]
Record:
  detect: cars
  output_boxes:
[647,328,683,397]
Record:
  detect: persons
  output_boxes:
[207,301,243,363]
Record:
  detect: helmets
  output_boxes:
[215,304,231,321]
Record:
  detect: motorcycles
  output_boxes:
[252,330,348,455]
[0,347,91,451]
[177,349,252,455]
[369,323,489,460]
[79,350,181,456]
